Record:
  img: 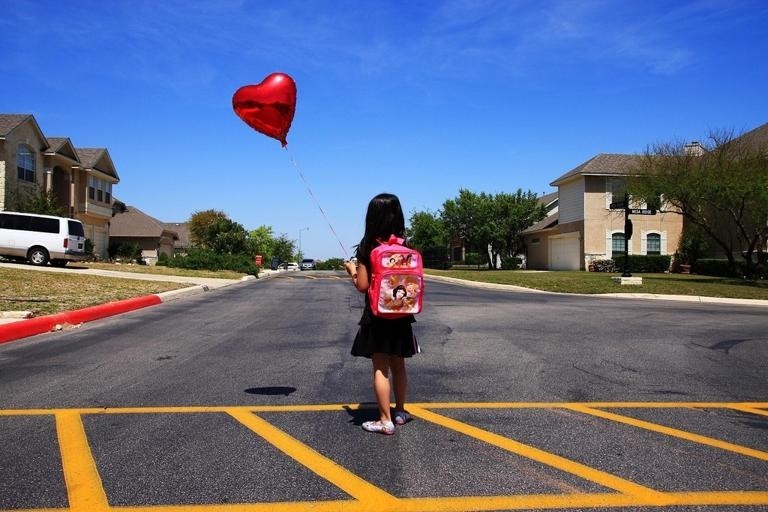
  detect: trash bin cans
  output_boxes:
[284,262,287,269]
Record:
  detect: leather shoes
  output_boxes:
[361,419,395,435]
[393,409,410,424]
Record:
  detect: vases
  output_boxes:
[680,264,691,274]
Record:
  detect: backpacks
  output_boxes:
[365,233,424,320]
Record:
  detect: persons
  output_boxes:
[381,253,421,312]
[344,192,423,434]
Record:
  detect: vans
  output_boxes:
[0,211,86,266]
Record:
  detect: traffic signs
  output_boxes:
[610,202,656,215]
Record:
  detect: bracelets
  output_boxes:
[352,274,357,283]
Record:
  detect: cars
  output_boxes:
[271,259,316,271]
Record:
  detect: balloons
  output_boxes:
[231,72,297,148]
